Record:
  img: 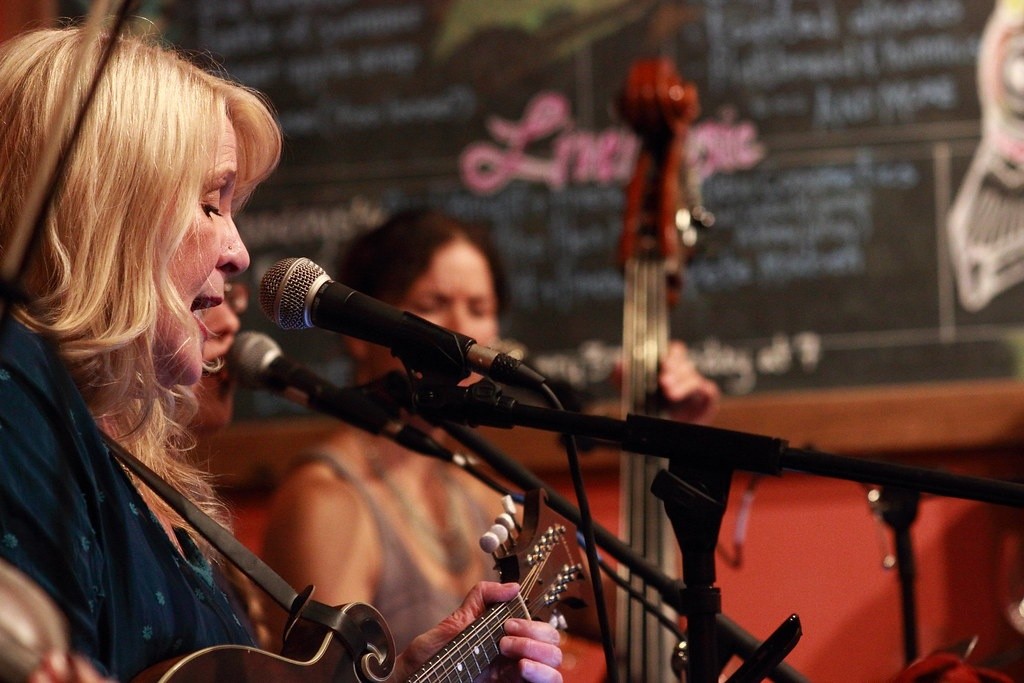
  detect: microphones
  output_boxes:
[231,335,454,461]
[259,257,546,391]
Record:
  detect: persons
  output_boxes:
[0,28,562,683]
[195,208,718,683]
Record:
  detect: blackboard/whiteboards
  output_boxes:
[41,0,1024,502]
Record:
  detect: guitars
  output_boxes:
[129,486,594,683]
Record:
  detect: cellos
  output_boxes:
[610,59,716,683]
